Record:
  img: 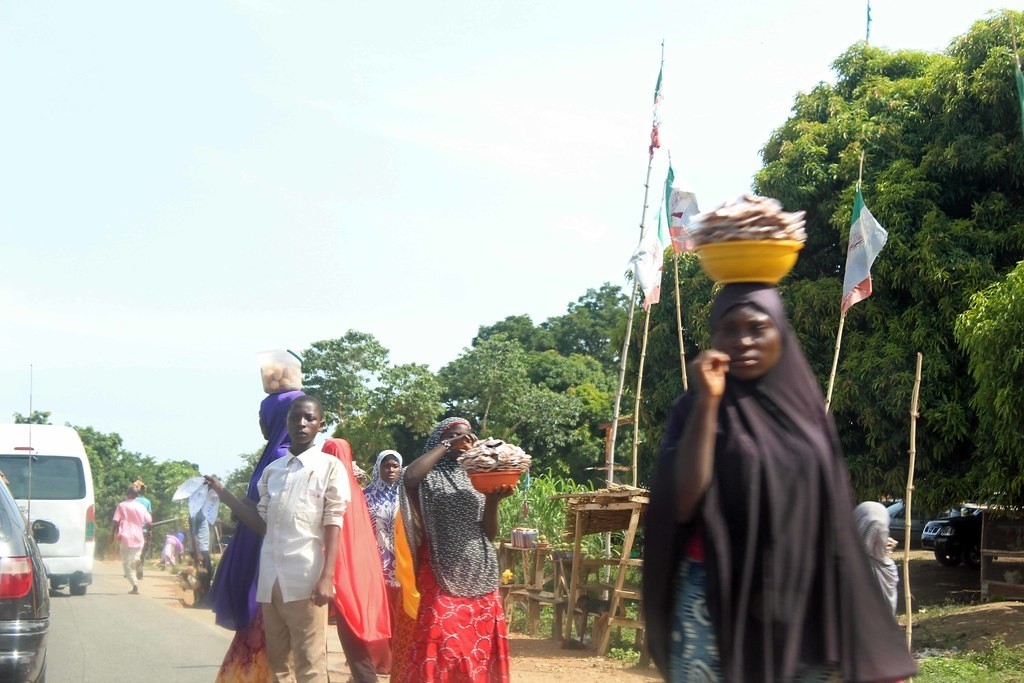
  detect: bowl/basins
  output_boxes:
[467,471,523,494]
[692,241,806,285]
[526,589,541,595]
[551,552,566,559]
[537,544,548,548]
[565,551,587,559]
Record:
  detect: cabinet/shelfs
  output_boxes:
[493,489,651,667]
[980,508,1024,601]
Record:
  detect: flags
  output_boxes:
[842,182,888,313]
[665,166,701,252]
[648,61,663,159]
[626,206,664,306]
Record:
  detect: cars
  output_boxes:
[880,491,1024,549]
[0,474,60,683]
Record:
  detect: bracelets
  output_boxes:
[439,440,452,452]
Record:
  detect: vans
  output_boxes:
[0,423,95,596]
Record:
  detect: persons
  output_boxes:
[109,479,152,593]
[640,281,921,683]
[189,509,213,601]
[204,391,351,683]
[363,416,513,683]
[161,531,183,570]
[320,438,391,683]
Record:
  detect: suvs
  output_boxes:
[921,509,1024,569]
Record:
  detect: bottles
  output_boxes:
[630,532,645,558]
[511,527,539,548]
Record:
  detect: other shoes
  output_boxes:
[128,586,138,595]
